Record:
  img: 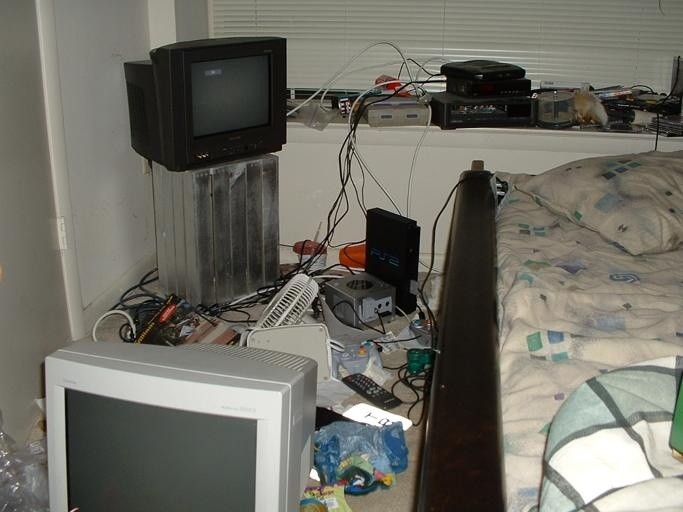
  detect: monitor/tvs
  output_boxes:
[44,339,319,512]
[124,36,288,172]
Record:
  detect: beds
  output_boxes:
[407,156,682,512]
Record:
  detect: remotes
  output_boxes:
[343,372,403,410]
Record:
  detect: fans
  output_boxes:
[254,274,321,327]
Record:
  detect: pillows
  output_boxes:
[510,149,682,258]
